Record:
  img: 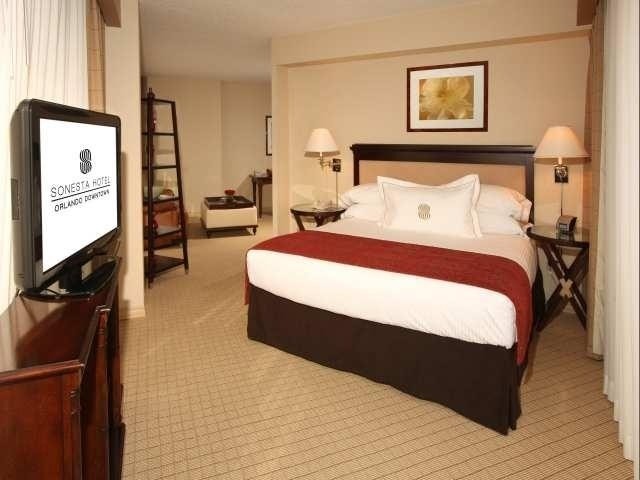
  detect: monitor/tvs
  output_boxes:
[20,98,122,299]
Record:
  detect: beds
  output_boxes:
[244,144,543,436]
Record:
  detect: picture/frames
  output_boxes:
[265,115,272,156]
[405,61,489,134]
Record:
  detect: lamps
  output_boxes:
[305,127,342,173]
[532,125,591,227]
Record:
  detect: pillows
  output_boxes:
[377,173,482,238]
[479,184,533,223]
[342,183,381,204]
[480,211,523,235]
[339,203,381,225]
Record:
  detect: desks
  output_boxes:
[527,224,595,331]
[291,202,346,231]
[250,174,273,217]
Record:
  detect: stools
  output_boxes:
[200,195,259,235]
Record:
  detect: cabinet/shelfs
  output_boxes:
[2,238,127,480]
[141,99,186,281]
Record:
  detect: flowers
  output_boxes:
[225,189,235,195]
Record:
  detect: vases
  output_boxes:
[227,195,232,199]
